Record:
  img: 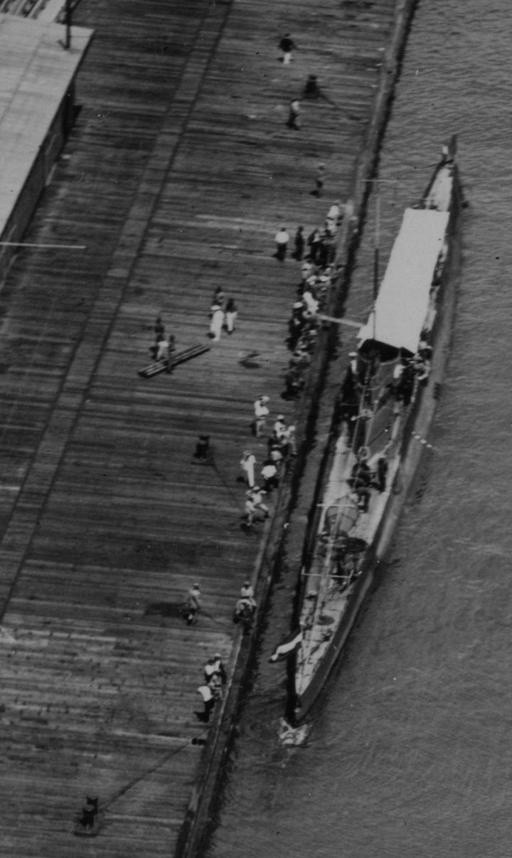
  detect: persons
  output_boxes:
[233,198,393,623]
[278,26,298,64]
[189,582,201,622]
[309,160,324,200]
[210,287,237,340]
[289,93,302,130]
[195,435,208,458]
[155,317,177,374]
[197,655,227,724]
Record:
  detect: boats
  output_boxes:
[276,131,468,763]
